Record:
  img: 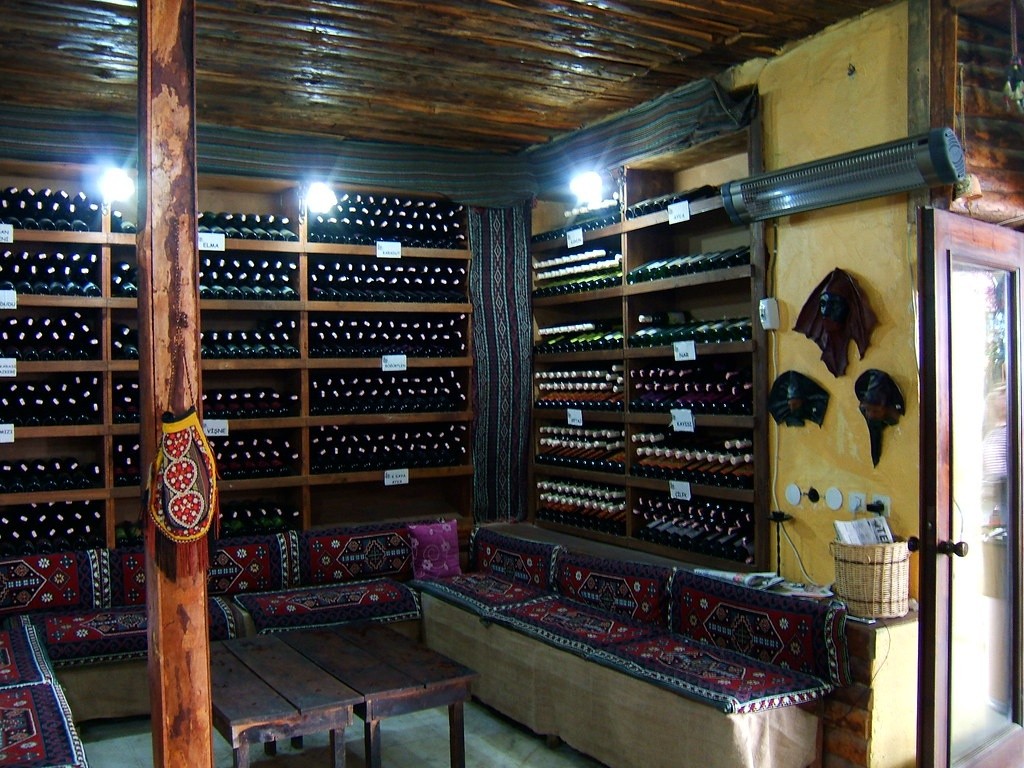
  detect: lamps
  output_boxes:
[569,168,614,202]
[298,177,338,214]
[720,126,966,224]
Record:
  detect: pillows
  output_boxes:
[409,518,461,580]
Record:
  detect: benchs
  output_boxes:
[409,522,850,768]
[0,519,407,768]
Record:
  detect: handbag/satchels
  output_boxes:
[139,323,221,584]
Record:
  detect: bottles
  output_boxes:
[195,181,759,567]
[0,180,144,558]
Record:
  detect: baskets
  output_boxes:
[826,534,911,619]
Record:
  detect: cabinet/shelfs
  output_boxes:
[0,203,473,547]
[525,121,770,573]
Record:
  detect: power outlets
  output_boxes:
[848,492,866,514]
[870,494,891,517]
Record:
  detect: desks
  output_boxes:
[825,607,919,768]
[210,618,481,768]
